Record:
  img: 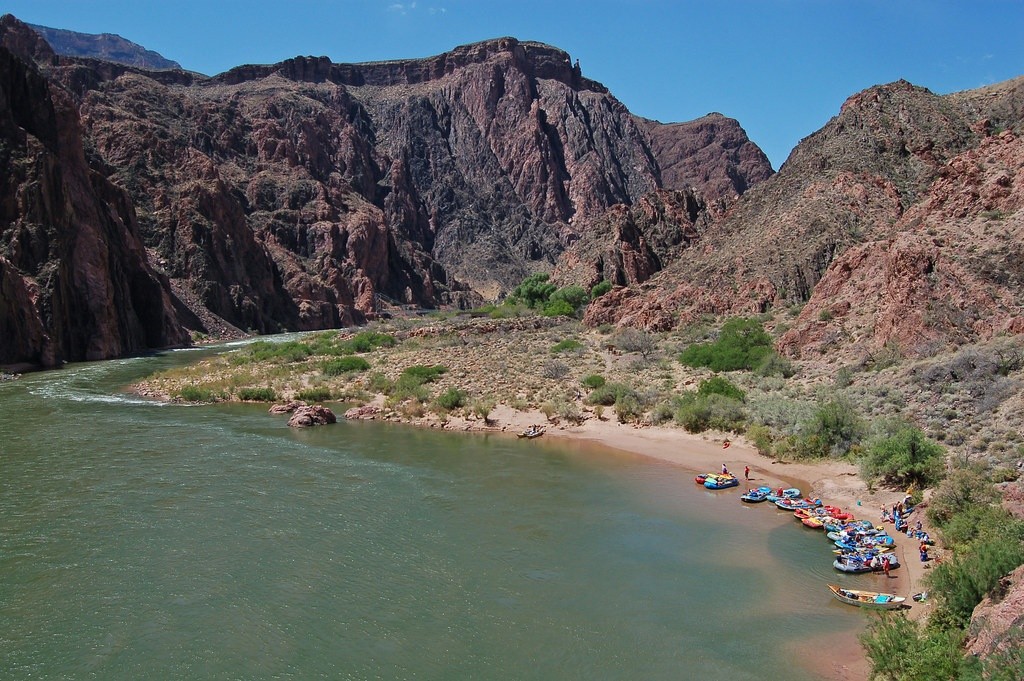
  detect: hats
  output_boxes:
[919,540,923,543]
[923,540,927,543]
[916,520,920,522]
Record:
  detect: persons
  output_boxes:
[870,555,879,574]
[881,501,931,562]
[883,557,890,575]
[776,487,783,496]
[744,465,749,480]
[722,464,728,474]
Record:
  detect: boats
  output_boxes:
[766,489,801,502]
[833,552,900,573]
[801,513,854,528]
[827,584,906,610]
[516,425,539,438]
[704,477,739,489]
[833,547,889,554]
[827,526,887,541]
[825,521,872,532]
[775,498,823,510]
[834,536,894,549]
[526,428,544,439]
[793,505,841,518]
[695,473,736,484]
[741,486,773,503]
